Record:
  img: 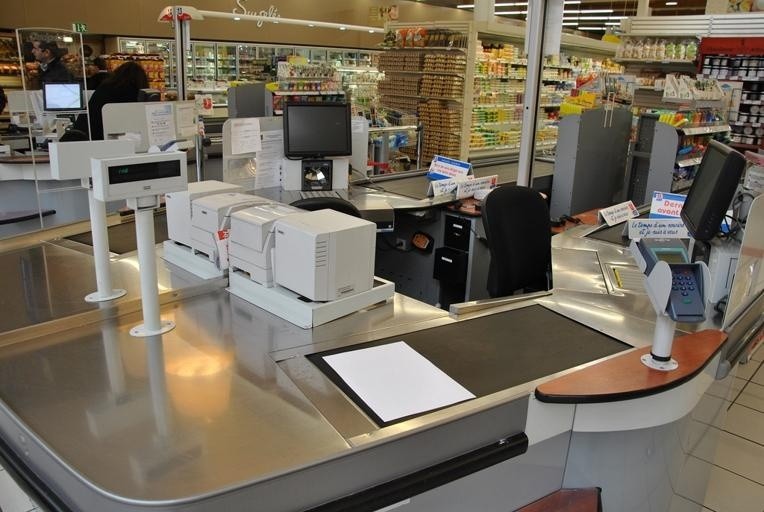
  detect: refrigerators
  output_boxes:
[104,36,386,91]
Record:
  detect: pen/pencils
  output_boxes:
[614,268,623,288]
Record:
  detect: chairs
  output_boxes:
[287,196,363,218]
[483,184,551,299]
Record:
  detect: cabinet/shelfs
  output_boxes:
[0,20,764,190]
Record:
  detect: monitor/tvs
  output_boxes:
[679,139,748,241]
[42,81,84,111]
[282,101,353,159]
[138,89,162,102]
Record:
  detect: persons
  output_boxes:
[58,61,150,142]
[31,39,73,89]
[82,58,111,90]
